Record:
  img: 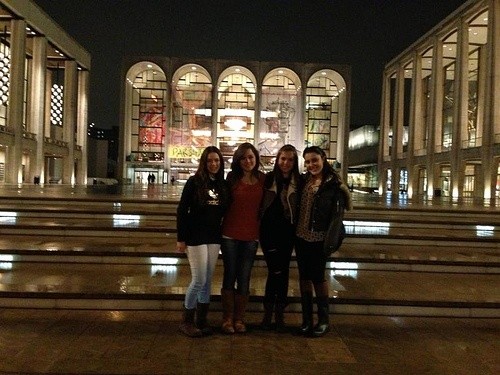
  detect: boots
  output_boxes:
[234,289,247,334]
[260,296,286,333]
[196,299,212,335]
[297,296,314,332]
[178,304,201,338]
[311,296,329,336]
[220,288,234,334]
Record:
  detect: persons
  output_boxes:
[148,174,155,184]
[258,145,300,330]
[220,142,265,333]
[292,147,352,336]
[177,147,226,337]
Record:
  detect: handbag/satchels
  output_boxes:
[334,217,346,252]
